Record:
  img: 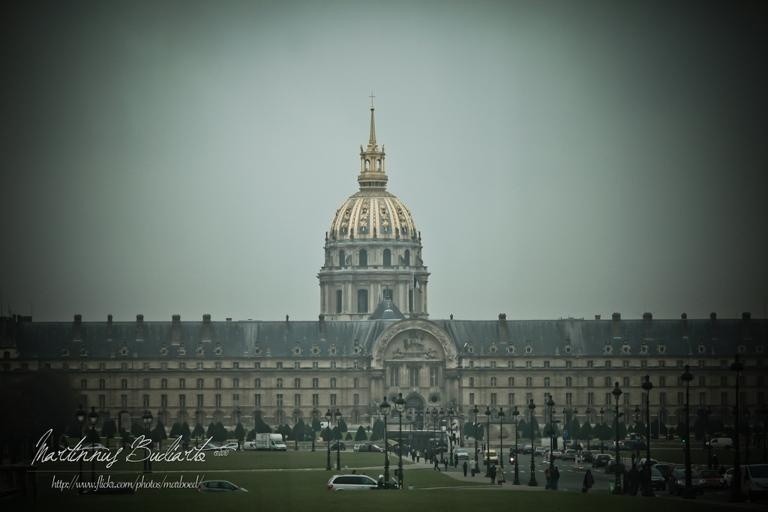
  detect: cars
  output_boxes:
[329,440,380,454]
[193,438,258,454]
[454,449,470,461]
[78,481,137,496]
[508,443,768,502]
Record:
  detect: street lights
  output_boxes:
[71,405,165,489]
[310,408,317,453]
[511,405,520,486]
[545,394,557,489]
[235,407,241,452]
[335,408,343,472]
[526,398,538,486]
[324,408,331,471]
[394,392,407,489]
[471,405,480,473]
[549,404,751,452]
[379,395,390,490]
[611,380,625,497]
[483,406,492,478]
[679,365,698,500]
[640,375,656,499]
[408,408,454,468]
[497,407,506,483]
[727,353,745,504]
[293,409,298,451]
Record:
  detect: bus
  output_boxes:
[384,428,449,458]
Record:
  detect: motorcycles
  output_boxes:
[370,477,401,491]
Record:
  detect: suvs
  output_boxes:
[196,479,251,494]
[326,474,380,493]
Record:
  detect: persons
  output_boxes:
[404,438,767,497]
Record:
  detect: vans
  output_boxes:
[482,449,499,466]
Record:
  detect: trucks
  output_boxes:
[243,431,290,452]
